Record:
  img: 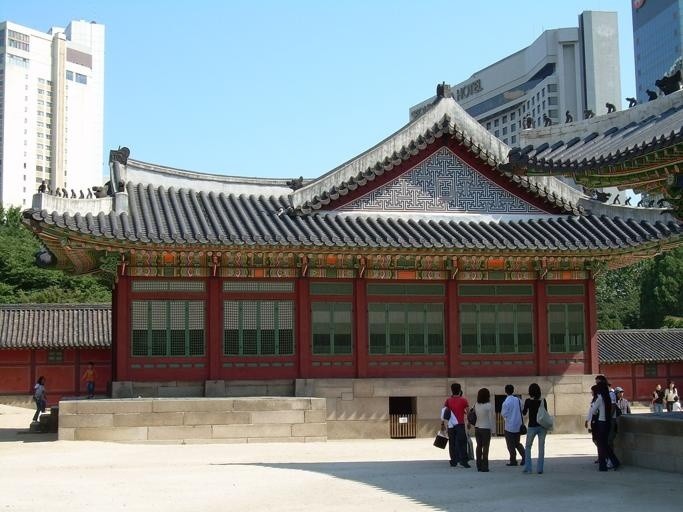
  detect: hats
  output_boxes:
[614,387,624,393]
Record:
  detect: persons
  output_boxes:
[652,384,666,413]
[665,380,678,411]
[523,383,547,474]
[459,391,470,438]
[472,388,497,472]
[441,382,471,468]
[585,374,619,472]
[614,386,631,415]
[32,376,48,422]
[82,361,98,399]
[671,396,681,411]
[501,384,528,466]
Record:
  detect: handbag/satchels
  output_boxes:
[537,407,553,431]
[520,424,527,435]
[467,408,477,426]
[467,438,474,460]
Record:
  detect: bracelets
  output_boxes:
[441,424,444,426]
